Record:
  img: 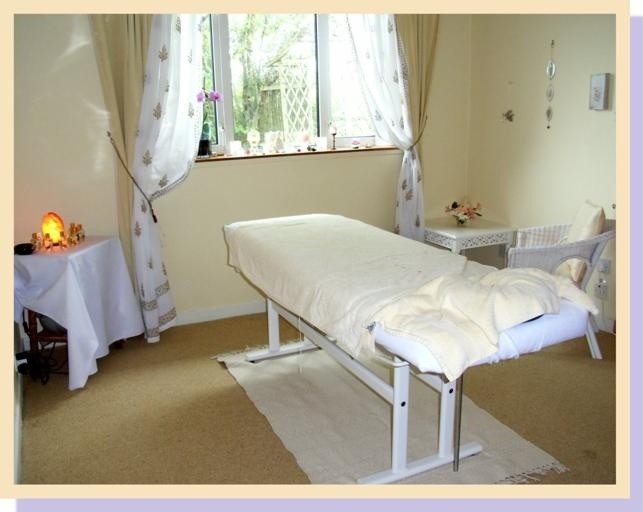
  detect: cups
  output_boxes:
[226,131,328,157]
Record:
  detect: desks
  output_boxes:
[14,235,144,392]
[420,214,518,268]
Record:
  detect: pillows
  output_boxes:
[562,200,605,245]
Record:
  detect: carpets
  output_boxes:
[212,336,570,484]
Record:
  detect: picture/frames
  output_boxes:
[589,73,609,110]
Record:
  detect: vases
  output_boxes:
[196,140,209,156]
[456,219,463,227]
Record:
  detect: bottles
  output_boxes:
[30,223,85,253]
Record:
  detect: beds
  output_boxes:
[224,214,588,484]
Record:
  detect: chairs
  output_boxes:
[507,218,616,361]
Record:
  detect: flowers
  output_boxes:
[196,76,223,140]
[442,200,483,223]
